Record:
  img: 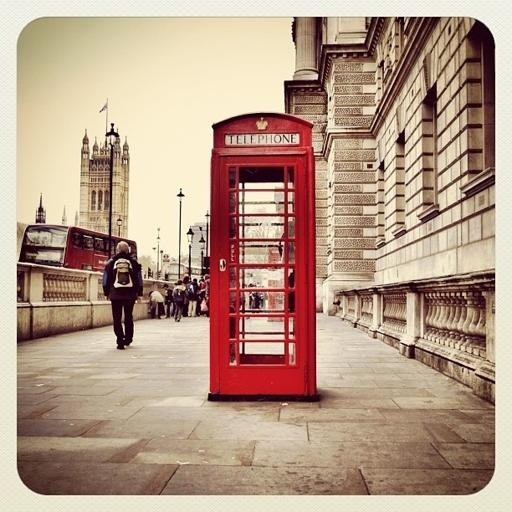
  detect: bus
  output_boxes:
[15,221,140,302]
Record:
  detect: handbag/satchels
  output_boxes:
[200,298,209,311]
[184,288,196,300]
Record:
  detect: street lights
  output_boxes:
[115,217,122,238]
[105,119,121,263]
[149,185,210,283]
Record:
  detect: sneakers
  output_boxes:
[117,345,125,350]
[125,338,132,345]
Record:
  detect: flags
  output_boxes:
[98,101,107,113]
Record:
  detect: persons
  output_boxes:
[148,272,263,322]
[101,240,145,350]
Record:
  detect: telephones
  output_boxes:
[278,221,295,264]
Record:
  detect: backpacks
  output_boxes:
[176,291,183,305]
[113,254,134,289]
